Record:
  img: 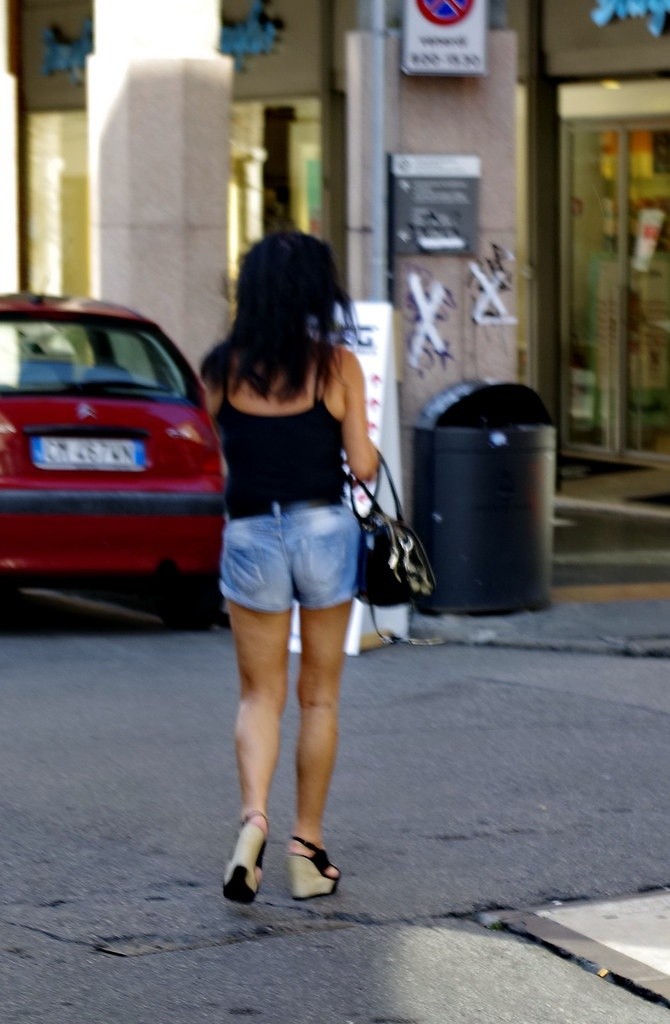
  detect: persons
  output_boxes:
[201,232,378,903]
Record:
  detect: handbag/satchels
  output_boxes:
[349,451,438,607]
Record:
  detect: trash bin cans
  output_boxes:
[411,376,555,615]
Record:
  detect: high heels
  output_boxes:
[286,835,341,901]
[222,810,270,905]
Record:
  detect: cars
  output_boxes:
[0,293,229,628]
